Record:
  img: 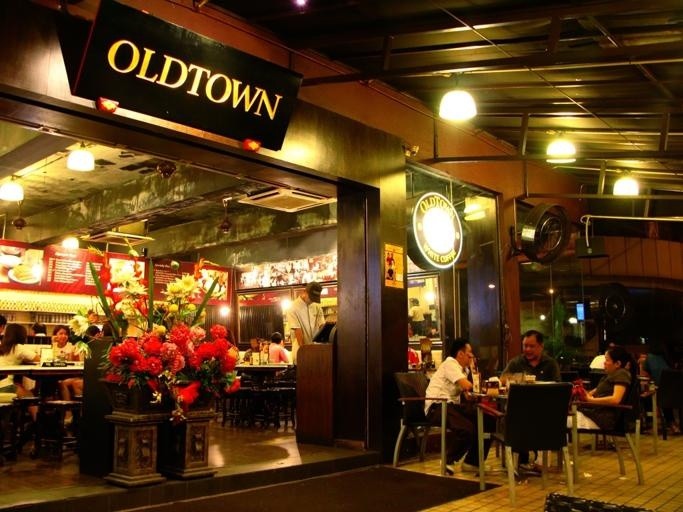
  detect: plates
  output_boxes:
[8,269,40,285]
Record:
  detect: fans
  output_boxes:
[510,203,572,265]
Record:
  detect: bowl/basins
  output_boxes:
[0,255,21,268]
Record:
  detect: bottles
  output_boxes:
[472,357,482,393]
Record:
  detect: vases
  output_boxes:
[98,378,218,488]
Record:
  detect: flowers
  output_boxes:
[66,237,242,427]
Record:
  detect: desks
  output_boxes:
[0,359,84,400]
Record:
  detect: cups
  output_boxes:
[486,381,499,397]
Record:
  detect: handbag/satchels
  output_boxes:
[0,378,17,404]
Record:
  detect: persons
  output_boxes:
[0,308,128,455]
[287,281,326,367]
[408,347,419,365]
[499,328,561,465]
[522,344,634,473]
[608,340,636,373]
[638,339,682,436]
[407,299,425,342]
[425,336,497,475]
[427,328,438,337]
[242,333,295,365]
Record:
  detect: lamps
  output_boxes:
[67,140,94,172]
[0,175,24,201]
[439,72,477,121]
[546,131,576,164]
[613,167,639,196]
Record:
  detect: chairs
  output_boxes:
[640,368,678,453]
[215,363,296,430]
[566,378,645,484]
[0,383,83,459]
[393,372,453,476]
[474,381,573,505]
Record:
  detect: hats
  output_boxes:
[306,281,322,302]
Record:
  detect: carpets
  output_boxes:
[115,463,503,512]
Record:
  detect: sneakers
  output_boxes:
[519,460,543,475]
[440,459,454,476]
[462,463,490,472]
